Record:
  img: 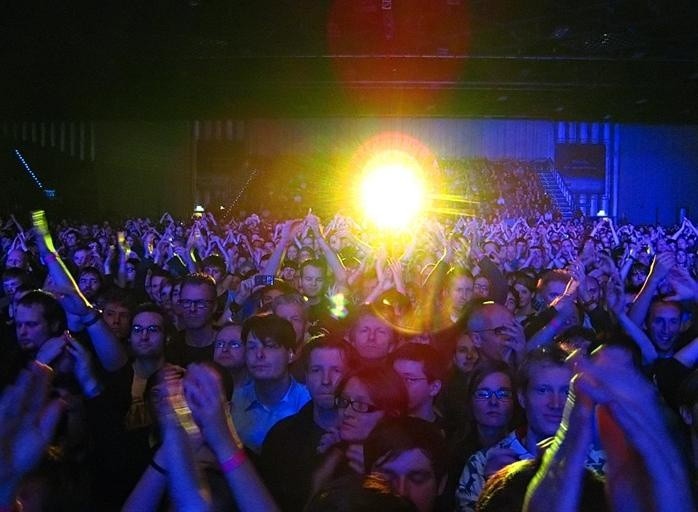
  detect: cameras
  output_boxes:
[255,275,274,285]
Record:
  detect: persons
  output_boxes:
[1,152,698,512]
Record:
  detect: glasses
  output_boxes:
[401,374,430,386]
[333,394,384,415]
[214,338,243,351]
[126,266,137,274]
[472,325,510,337]
[130,325,167,337]
[177,298,215,310]
[472,386,516,401]
